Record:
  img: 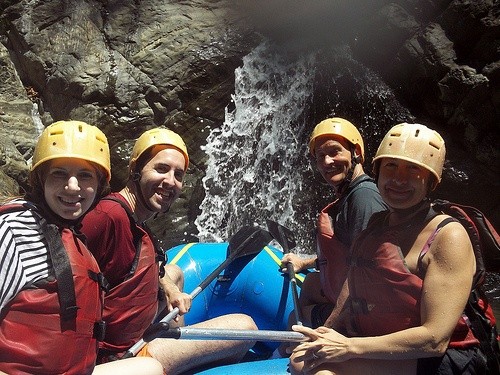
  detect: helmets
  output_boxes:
[127,126,189,175]
[371,122,448,188]
[309,117,365,163]
[27,120,112,187]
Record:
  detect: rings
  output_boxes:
[313,353,320,359]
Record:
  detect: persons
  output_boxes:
[0,120,164,375]
[288,123,490,375]
[79,128,258,375]
[263,116,390,360]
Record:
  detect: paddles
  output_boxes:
[265,218,306,325]
[142,322,312,342]
[119,226,274,360]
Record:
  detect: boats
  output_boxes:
[157,241,318,375]
